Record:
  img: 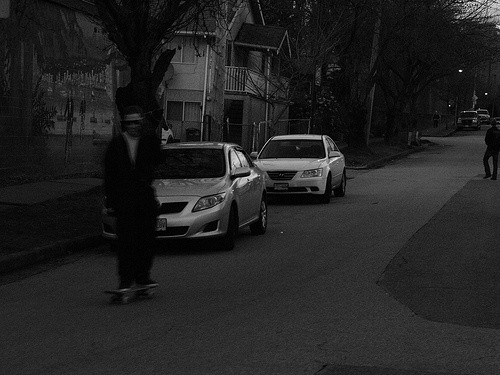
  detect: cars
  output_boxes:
[249,134,347,204]
[99,141,268,251]
[491,117,500,124]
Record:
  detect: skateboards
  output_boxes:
[102,282,158,304]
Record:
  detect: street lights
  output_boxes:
[265,48,280,141]
[200,31,217,142]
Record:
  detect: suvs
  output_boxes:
[476,109,490,125]
[457,111,480,131]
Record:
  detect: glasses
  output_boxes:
[123,119,143,125]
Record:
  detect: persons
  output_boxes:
[102,105,162,289]
[483,120,500,180]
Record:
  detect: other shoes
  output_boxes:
[135,278,158,288]
[490,178,497,180]
[119,280,135,291]
[483,175,491,178]
